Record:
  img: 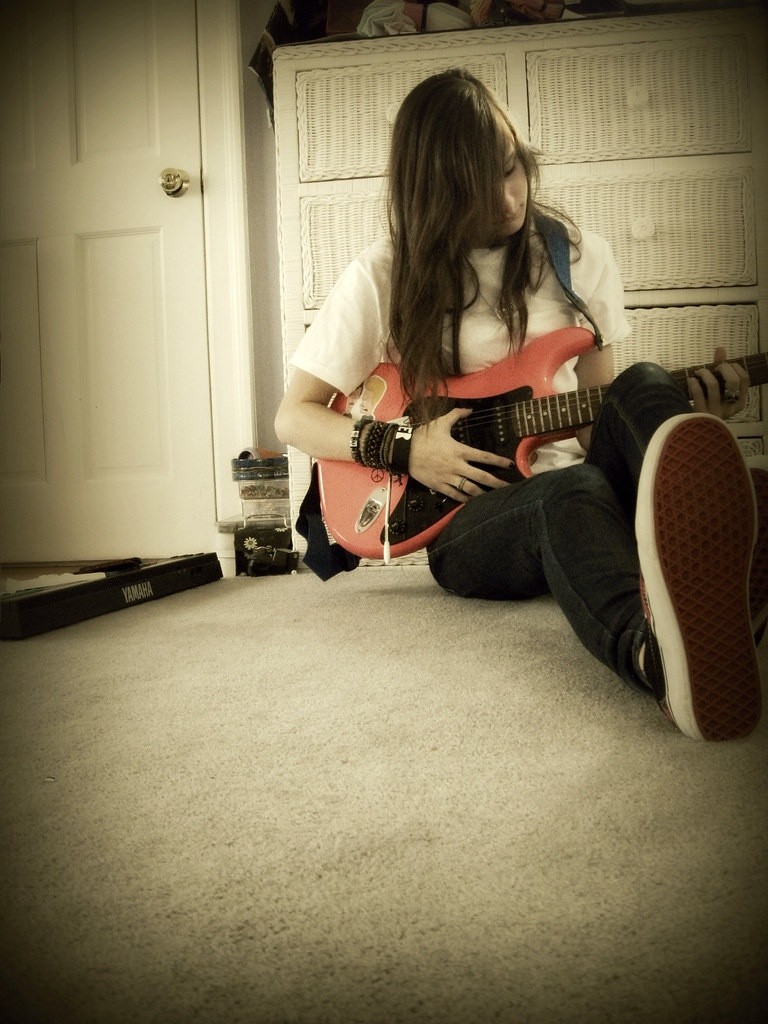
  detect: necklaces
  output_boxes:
[462,253,522,323]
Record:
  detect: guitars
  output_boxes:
[316,326,768,560]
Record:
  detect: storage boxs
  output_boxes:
[218,479,293,577]
[326,0,423,32]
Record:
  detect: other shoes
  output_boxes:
[635,414,768,743]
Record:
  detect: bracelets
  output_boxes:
[350,419,413,474]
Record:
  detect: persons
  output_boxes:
[273,65,768,745]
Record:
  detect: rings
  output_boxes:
[726,389,739,403]
[458,477,467,490]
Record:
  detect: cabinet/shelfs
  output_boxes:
[270,12,768,575]
